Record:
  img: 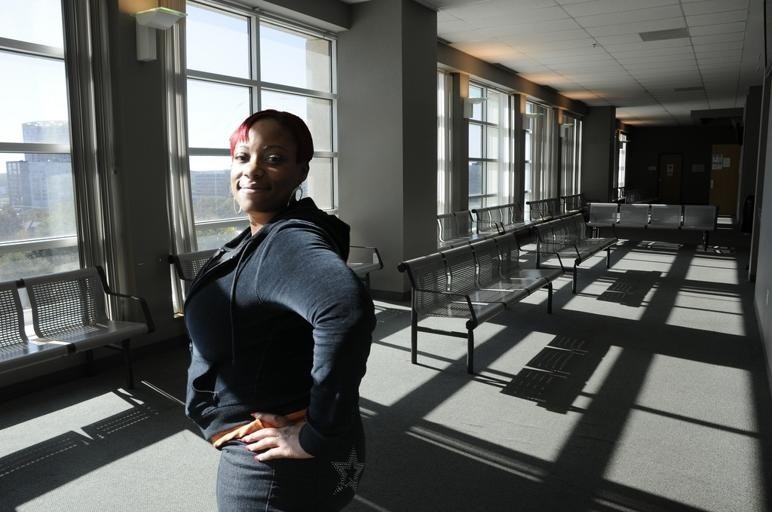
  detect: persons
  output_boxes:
[181,108,375,510]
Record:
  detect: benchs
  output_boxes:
[398,231,566,375]
[588,201,615,240]
[534,214,615,297]
[437,194,586,276]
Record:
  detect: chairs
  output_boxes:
[615,203,718,252]
[159,245,382,327]
[0,265,156,394]
